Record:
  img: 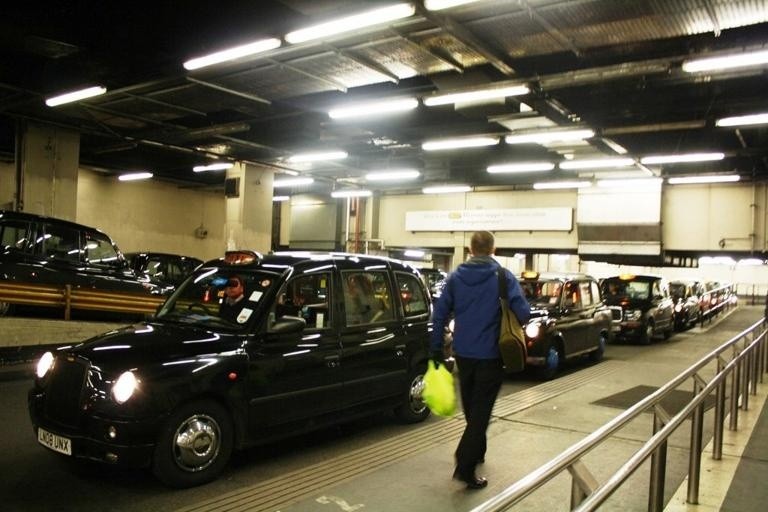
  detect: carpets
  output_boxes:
[588,383,727,421]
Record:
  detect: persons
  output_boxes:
[350,274,377,305]
[431,229,529,486]
[221,276,248,322]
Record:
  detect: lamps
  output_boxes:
[558,156,637,172]
[284,147,349,165]
[485,159,555,175]
[503,128,597,145]
[532,178,594,190]
[594,175,664,187]
[270,194,291,203]
[270,175,316,189]
[328,189,374,199]
[638,149,727,165]
[422,82,528,109]
[365,169,420,182]
[680,49,767,73]
[421,134,501,151]
[117,170,154,181]
[328,97,418,121]
[180,36,281,71]
[420,182,475,197]
[708,110,767,128]
[191,161,234,173]
[283,4,415,45]
[666,172,740,187]
[44,83,107,107]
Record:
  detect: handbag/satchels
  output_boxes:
[421,360,457,416]
[499,307,527,372]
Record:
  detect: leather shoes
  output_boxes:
[453,469,487,488]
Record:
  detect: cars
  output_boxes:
[90,253,208,297]
[443,269,613,376]
[599,275,678,347]
[25,251,454,489]
[394,255,451,303]
[0,208,161,323]
[665,280,732,330]
